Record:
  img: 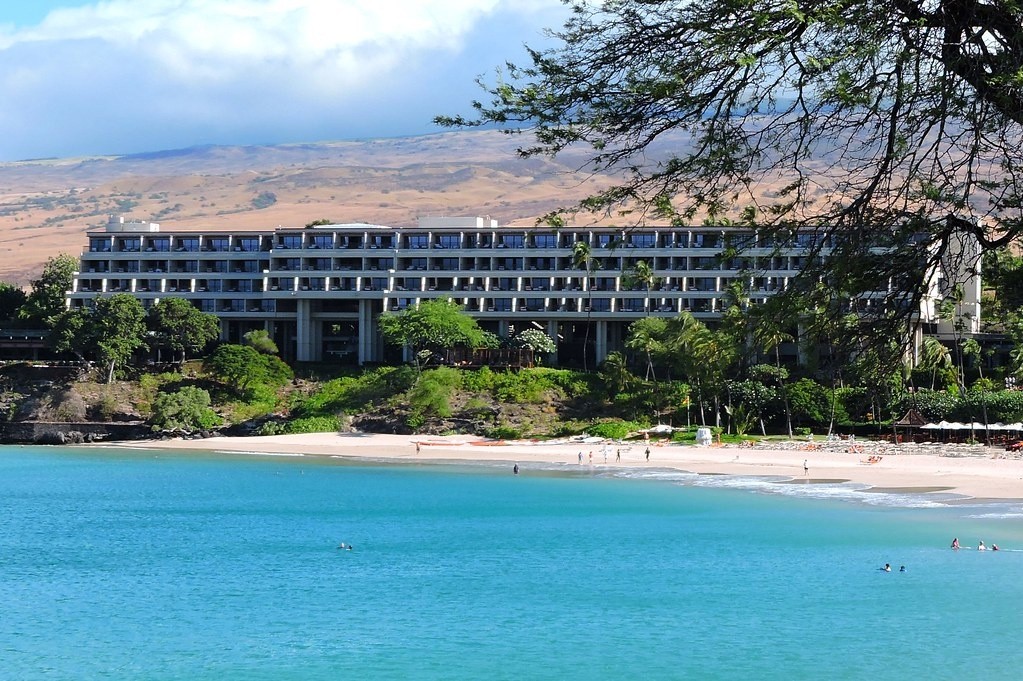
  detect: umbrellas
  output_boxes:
[920,421,1023,441]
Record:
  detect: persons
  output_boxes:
[804,459,809,475]
[417,442,420,454]
[578,452,583,464]
[978,541,984,550]
[885,563,891,571]
[514,463,519,475]
[645,447,650,462]
[604,451,607,463]
[992,544,998,550]
[900,566,906,571]
[589,452,593,463]
[952,538,959,548]
[616,450,620,462]
